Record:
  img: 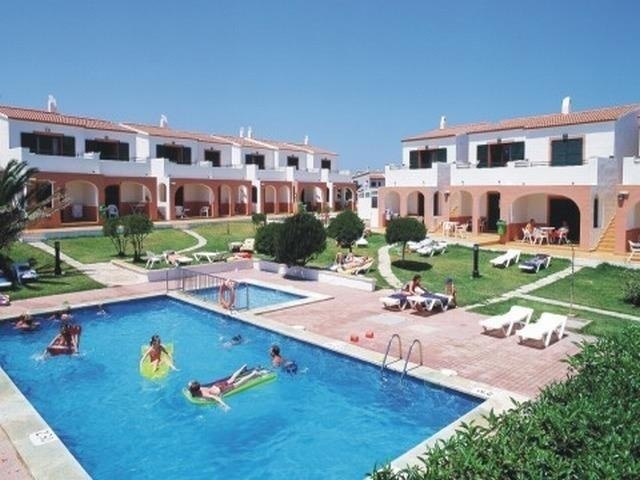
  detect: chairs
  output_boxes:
[406,215,570,275]
[515,311,568,348]
[380,283,455,314]
[626,240,640,262]
[329,254,376,278]
[479,305,534,337]
[353,237,369,248]
[143,236,257,271]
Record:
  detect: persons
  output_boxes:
[218,334,242,352]
[269,347,284,368]
[336,218,569,310]
[0,294,108,356]
[188,364,262,411]
[139,335,180,374]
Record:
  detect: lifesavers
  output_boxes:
[218,281,236,309]
